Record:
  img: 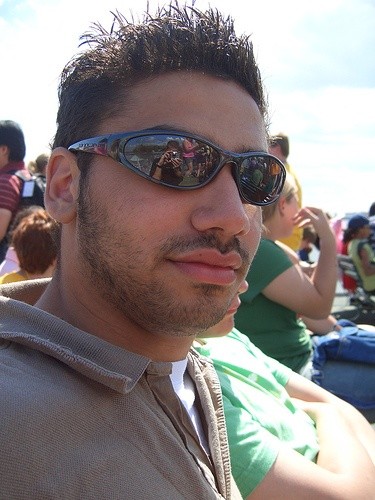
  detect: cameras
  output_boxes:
[172,151,177,158]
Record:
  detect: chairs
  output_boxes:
[336,253,375,323]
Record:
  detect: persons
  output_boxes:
[248,156,282,193]
[194,280,375,500]
[234,172,375,409]
[0,0,286,500]
[268,135,303,254]
[0,119,60,283]
[151,137,215,185]
[300,215,375,292]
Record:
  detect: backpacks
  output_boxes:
[9,169,47,208]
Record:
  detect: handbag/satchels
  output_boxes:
[311,318,375,364]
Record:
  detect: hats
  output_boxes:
[349,215,370,232]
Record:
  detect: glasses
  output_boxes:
[68,129,288,206]
